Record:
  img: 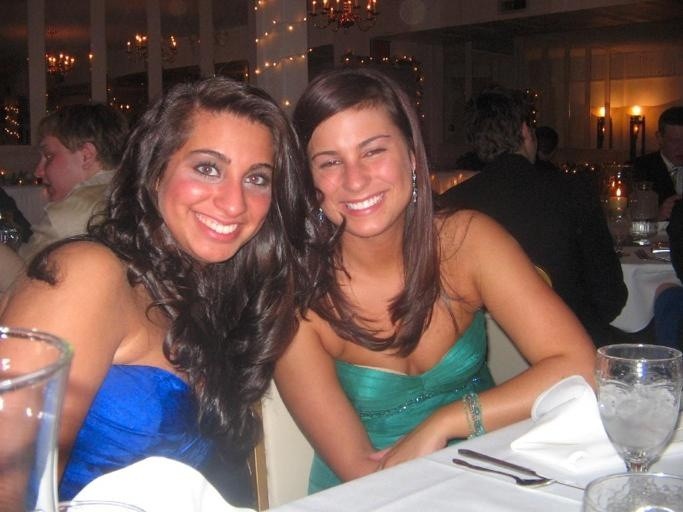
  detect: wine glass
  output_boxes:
[594,342,682,511]
[608,210,630,258]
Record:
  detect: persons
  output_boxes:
[17,102,132,266]
[0,75,318,511]
[272,69,611,495]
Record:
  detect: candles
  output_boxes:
[608,188,627,212]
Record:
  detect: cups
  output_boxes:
[0,325,73,512]
[582,470,683,512]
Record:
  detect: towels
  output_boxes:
[66,457,257,512]
[507,372,629,490]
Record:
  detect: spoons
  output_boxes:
[451,457,556,489]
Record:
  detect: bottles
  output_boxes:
[629,183,658,238]
[0,212,23,248]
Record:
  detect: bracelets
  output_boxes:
[462,391,485,440]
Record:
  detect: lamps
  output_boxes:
[306,0,378,33]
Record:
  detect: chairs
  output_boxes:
[260,378,316,512]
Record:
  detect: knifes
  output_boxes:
[457,447,587,490]
